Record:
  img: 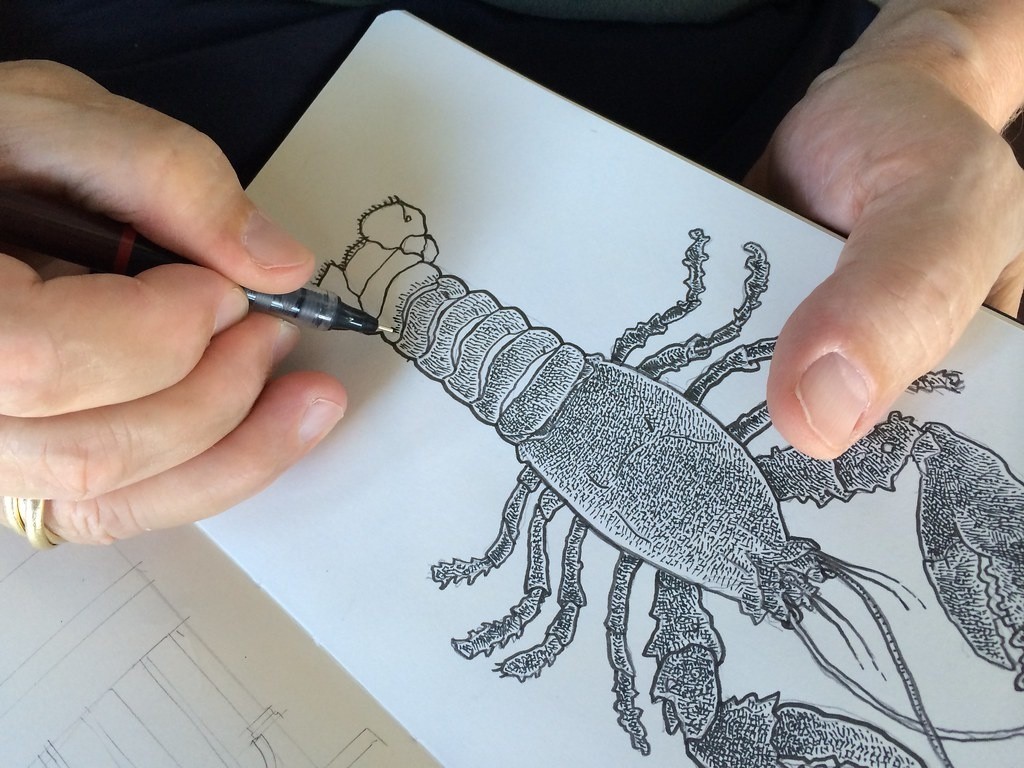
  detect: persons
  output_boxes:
[0,0,1024,550]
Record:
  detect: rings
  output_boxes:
[3,495,69,550]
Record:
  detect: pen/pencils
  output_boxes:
[0,180,403,336]
